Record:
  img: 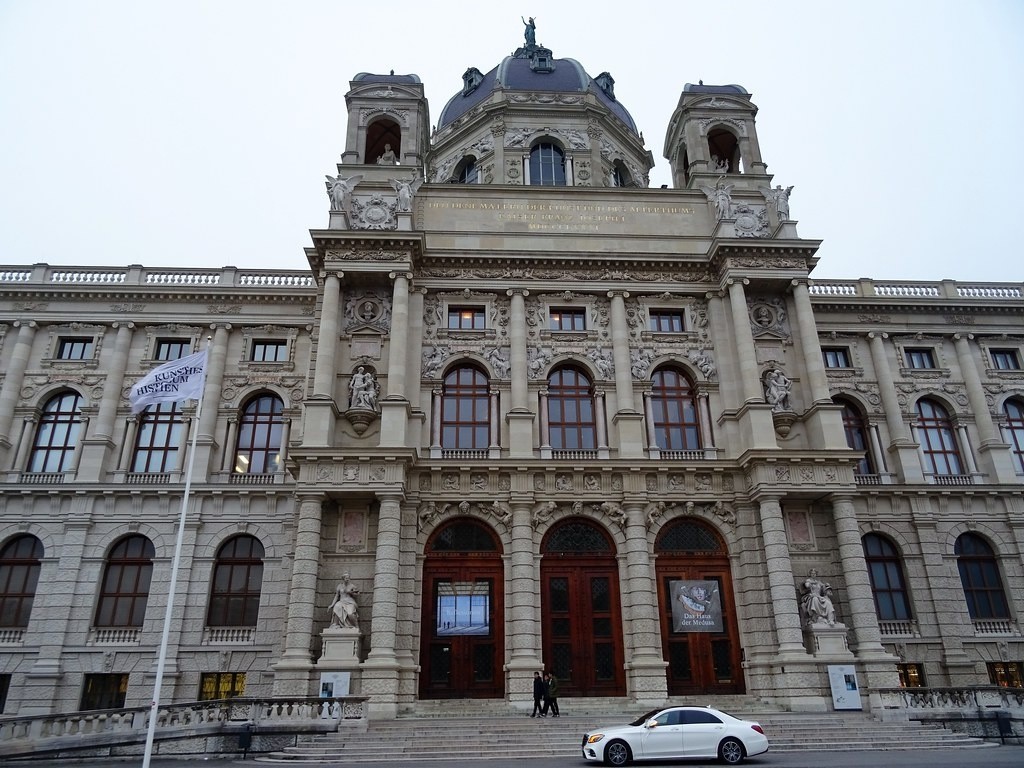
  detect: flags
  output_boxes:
[129,348,208,415]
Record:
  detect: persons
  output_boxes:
[529,344,545,378]
[423,340,446,377]
[692,347,716,378]
[539,672,559,717]
[765,370,792,412]
[756,307,770,326]
[770,184,790,219]
[377,143,396,165]
[326,174,414,212]
[711,155,729,173]
[715,182,731,217]
[530,671,543,717]
[490,344,507,377]
[800,568,835,625]
[591,345,611,378]
[521,16,537,47]
[348,367,377,412]
[326,573,360,630]
[363,301,374,315]
[632,346,651,375]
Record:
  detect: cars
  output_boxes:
[582,704,771,766]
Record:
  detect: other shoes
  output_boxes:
[530,714,561,718]
